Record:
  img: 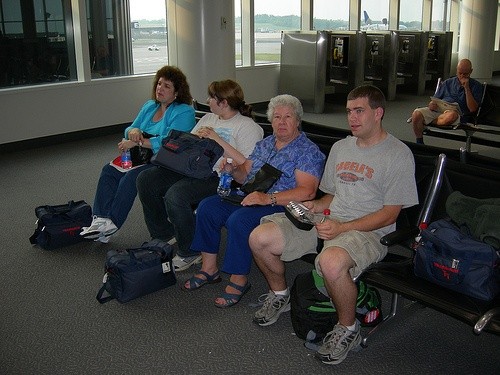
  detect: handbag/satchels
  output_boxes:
[97,244,177,306]
[414,221,500,302]
[153,127,225,181]
[242,162,281,195]
[129,132,158,163]
[29,200,93,251]
[287,270,385,341]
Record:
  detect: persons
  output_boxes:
[182,94,326,308]
[249,84,420,365]
[80,67,196,244]
[412,59,483,144]
[136,81,264,271]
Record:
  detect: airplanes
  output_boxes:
[340,11,407,32]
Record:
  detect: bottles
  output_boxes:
[320,209,333,224]
[120,148,131,169]
[217,157,232,197]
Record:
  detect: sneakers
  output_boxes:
[79,215,119,245]
[253,292,292,327]
[315,318,362,365]
[164,235,176,247]
[172,250,202,271]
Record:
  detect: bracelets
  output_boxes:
[270,194,276,206]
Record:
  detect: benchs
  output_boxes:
[407,78,500,153]
[192,100,500,349]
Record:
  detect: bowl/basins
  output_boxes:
[284,200,316,231]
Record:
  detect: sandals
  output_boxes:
[215,279,251,309]
[181,266,221,291]
